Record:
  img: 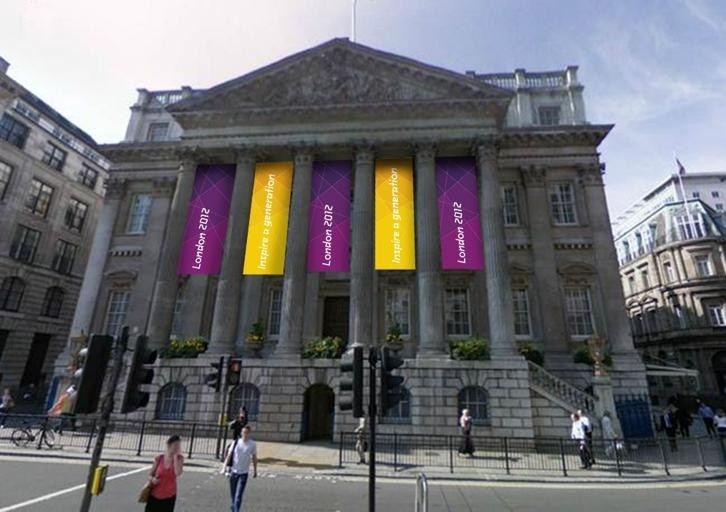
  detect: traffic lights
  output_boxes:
[380,341,405,417]
[68,333,113,416]
[90,464,108,495]
[118,334,158,413]
[206,355,224,392]
[339,342,364,418]
[226,358,242,385]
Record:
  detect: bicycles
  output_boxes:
[10,416,56,447]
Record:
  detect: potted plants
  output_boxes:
[243,315,271,360]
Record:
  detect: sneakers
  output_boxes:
[356,458,366,465]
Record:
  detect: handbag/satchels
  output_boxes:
[137,477,154,504]
[225,444,234,467]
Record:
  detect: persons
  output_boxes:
[576,408,595,467]
[569,411,593,471]
[456,408,478,461]
[354,417,369,465]
[228,404,248,440]
[655,392,726,460]
[600,410,620,459]
[218,424,258,512]
[0,381,79,436]
[144,434,185,512]
